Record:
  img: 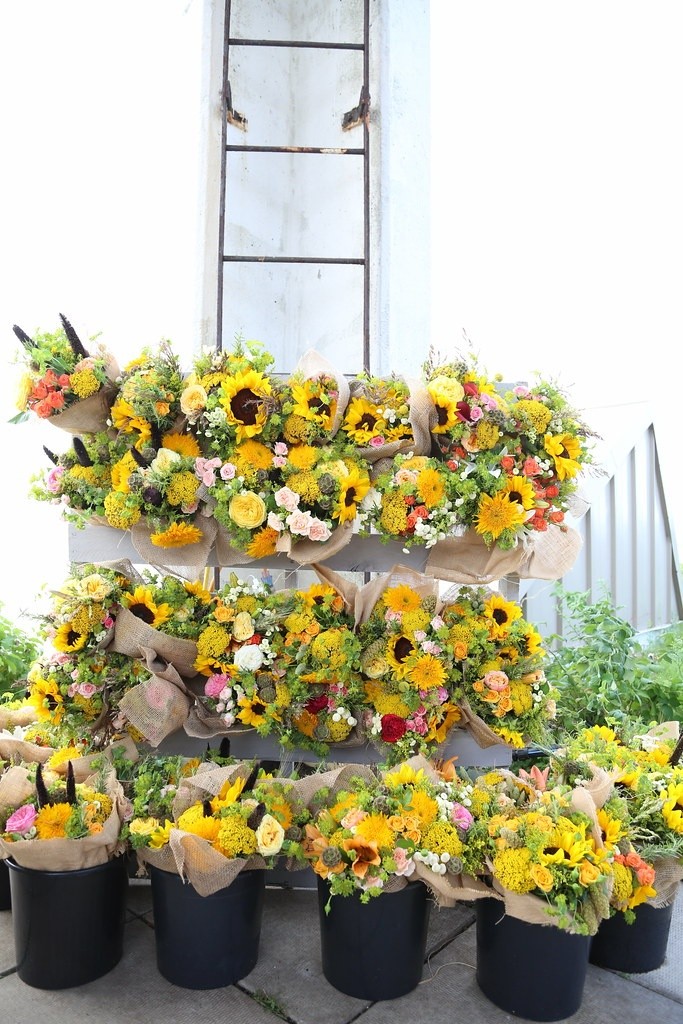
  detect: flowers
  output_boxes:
[9,312,109,426]
[555,728,682,855]
[194,444,369,556]
[337,368,415,445]
[304,766,463,914]
[356,582,460,758]
[195,575,291,737]
[30,566,128,752]
[470,769,615,937]
[0,761,130,863]
[117,577,216,644]
[446,587,559,746]
[110,342,186,448]
[182,344,284,443]
[105,433,202,549]
[34,437,118,530]
[359,452,480,555]
[129,755,295,873]
[424,348,602,479]
[468,457,573,550]
[279,585,365,754]
[264,377,337,445]
